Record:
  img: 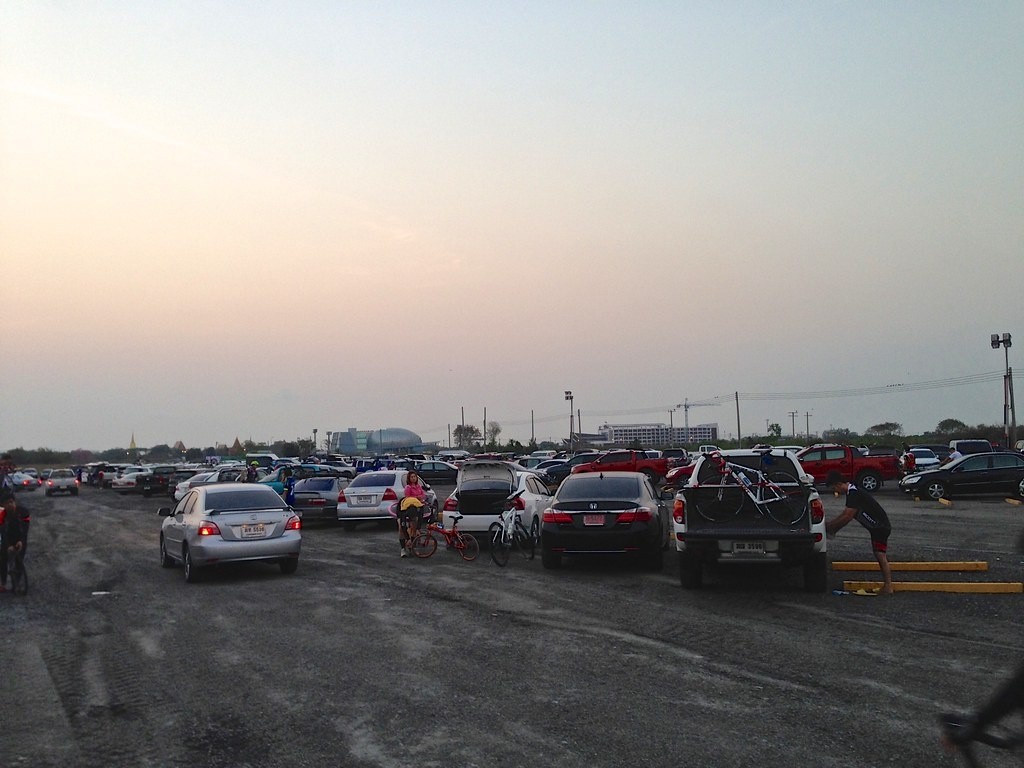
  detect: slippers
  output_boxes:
[870,587,891,595]
[852,589,877,596]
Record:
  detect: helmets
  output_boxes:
[251,461,259,466]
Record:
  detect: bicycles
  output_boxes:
[693,447,809,526]
[485,490,536,567]
[6,547,29,597]
[411,503,480,562]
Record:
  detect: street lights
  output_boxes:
[313,428,318,442]
[991,332,1013,449]
[564,390,575,453]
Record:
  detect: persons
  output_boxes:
[825,472,893,594]
[281,468,295,512]
[995,444,1004,452]
[404,471,433,546]
[664,455,678,472]
[0,492,30,592]
[940,447,962,464]
[396,497,424,558]
[406,461,418,471]
[903,446,916,477]
[388,461,396,470]
[248,464,259,482]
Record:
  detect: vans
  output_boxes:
[951,440,995,456]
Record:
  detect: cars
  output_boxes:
[24,468,80,497]
[899,451,1024,502]
[441,459,559,548]
[540,471,676,571]
[449,447,808,480]
[72,452,461,529]
[4,471,42,492]
[897,449,943,472]
[156,484,302,584]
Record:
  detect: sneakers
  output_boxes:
[401,549,408,557]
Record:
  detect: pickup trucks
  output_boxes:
[673,449,829,594]
[795,445,904,493]
[570,450,676,486]
